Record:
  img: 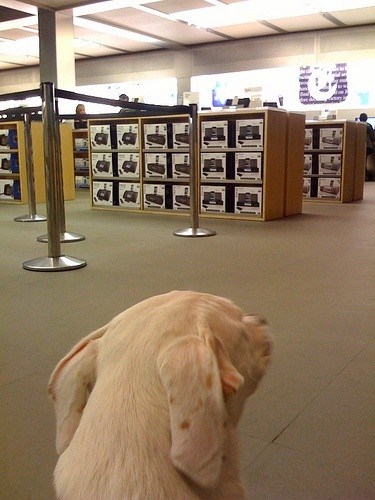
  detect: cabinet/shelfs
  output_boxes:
[0,106,366,223]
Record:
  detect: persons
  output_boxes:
[74,103,87,129]
[359,112,375,182]
[119,94,139,113]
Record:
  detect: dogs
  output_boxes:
[48,291,271,500]
